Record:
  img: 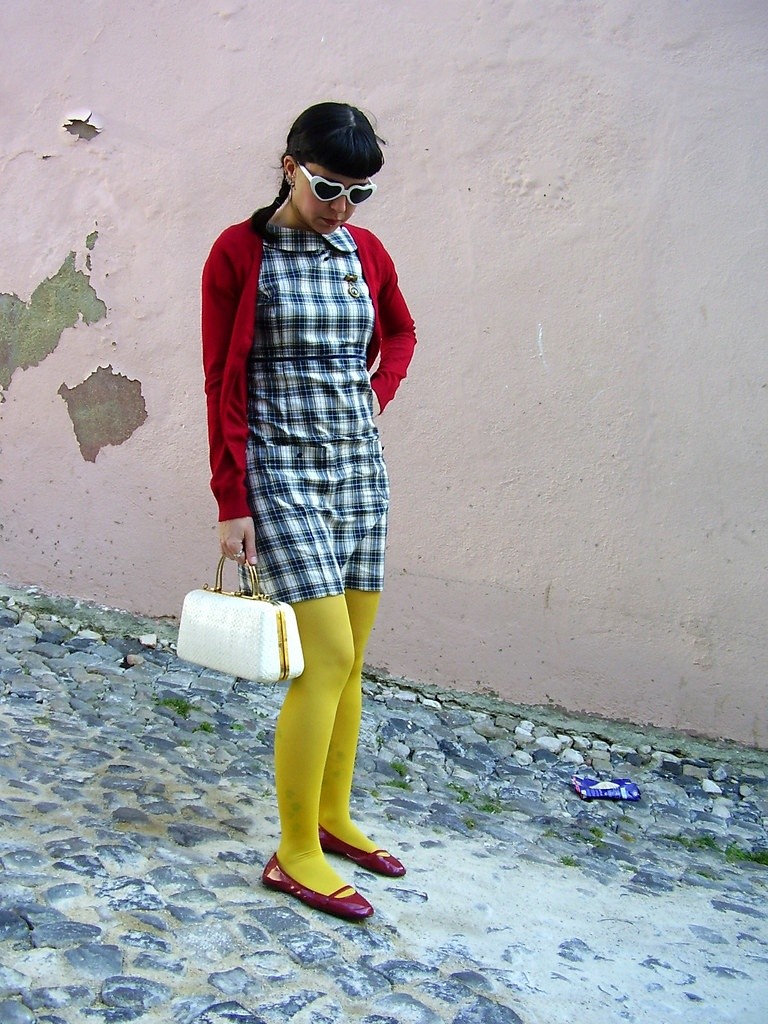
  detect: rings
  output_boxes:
[233,551,243,559]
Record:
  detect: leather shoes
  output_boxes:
[319,822,406,877]
[262,852,374,920]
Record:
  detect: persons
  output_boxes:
[201,102,417,921]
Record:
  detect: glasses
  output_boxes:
[296,161,378,206]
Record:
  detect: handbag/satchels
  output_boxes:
[176,553,305,684]
